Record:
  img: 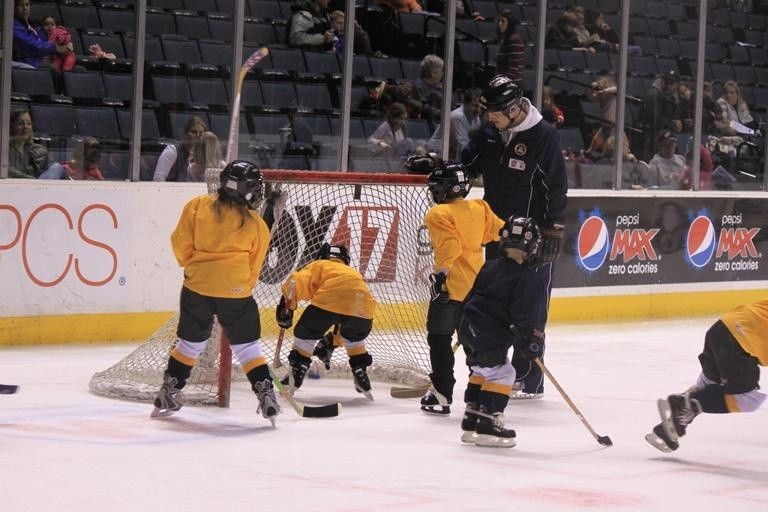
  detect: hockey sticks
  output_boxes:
[226,47,269,164]
[390,341,461,399]
[273,280,296,378]
[535,359,612,446]
[269,371,341,418]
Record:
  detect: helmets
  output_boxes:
[314,242,351,268]
[478,72,523,117]
[499,214,541,258]
[426,160,470,205]
[219,160,263,211]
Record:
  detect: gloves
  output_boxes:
[515,328,546,361]
[428,269,450,306]
[276,295,294,329]
[314,331,339,369]
[529,220,567,263]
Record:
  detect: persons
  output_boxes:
[9,0,768,193]
[420,159,505,407]
[153,159,284,418]
[458,215,546,439]
[275,242,377,394]
[652,299,768,450]
[403,72,570,395]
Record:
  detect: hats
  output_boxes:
[660,67,681,82]
[656,128,679,144]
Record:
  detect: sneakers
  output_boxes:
[653,423,679,450]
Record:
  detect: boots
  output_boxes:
[460,389,479,431]
[512,361,546,394]
[668,393,702,438]
[349,357,371,394]
[250,371,280,418]
[153,367,182,412]
[280,354,311,387]
[476,394,517,438]
[419,371,457,405]
